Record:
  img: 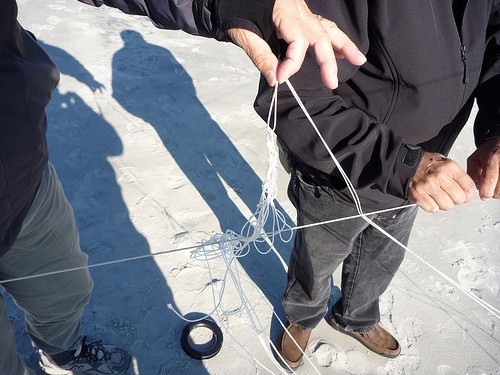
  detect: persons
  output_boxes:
[0,0,367,375]
[254,0,500,372]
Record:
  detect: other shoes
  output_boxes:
[329,306,401,359]
[276,321,312,371]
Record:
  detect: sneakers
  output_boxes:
[38,335,131,375]
